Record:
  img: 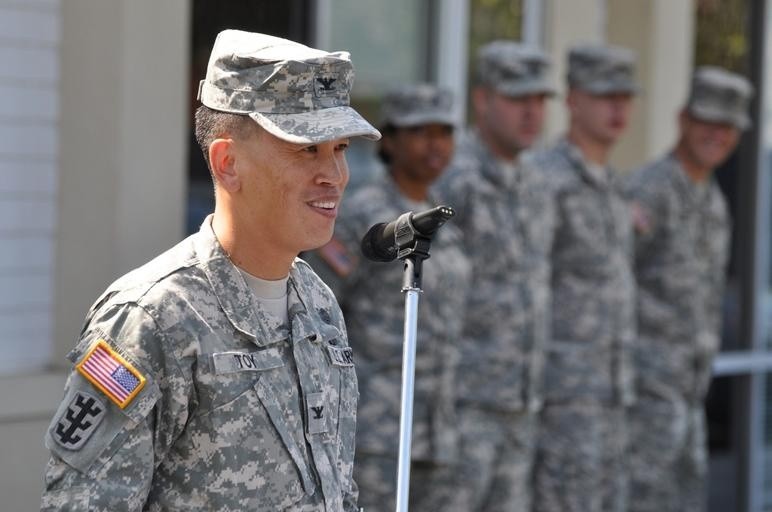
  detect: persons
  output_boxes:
[538,48,639,511]
[302,84,473,512]
[436,40,562,512]
[41,26,381,512]
[626,68,756,512]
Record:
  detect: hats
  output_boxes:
[478,41,555,96]
[692,65,753,130]
[197,29,382,145]
[569,44,643,95]
[388,82,459,127]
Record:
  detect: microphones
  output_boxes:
[361,204,456,263]
